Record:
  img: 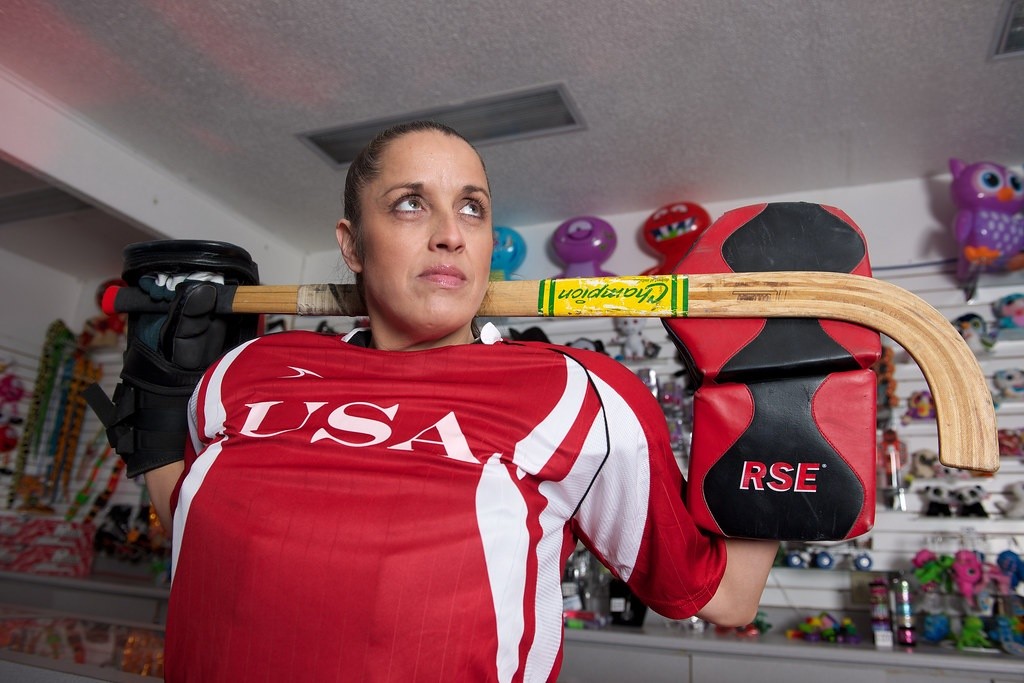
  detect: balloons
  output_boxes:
[484,223,527,285]
[545,217,621,278]
[639,203,716,276]
[943,152,1024,290]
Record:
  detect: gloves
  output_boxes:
[659,201,883,543]
[84,239,265,480]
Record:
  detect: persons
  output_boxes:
[82,119,885,683]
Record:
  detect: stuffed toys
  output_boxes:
[609,315,654,358]
[874,292,1024,606]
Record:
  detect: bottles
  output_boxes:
[876,429,901,489]
[868,578,892,642]
[896,578,918,646]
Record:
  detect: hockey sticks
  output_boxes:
[96,270,1002,472]
